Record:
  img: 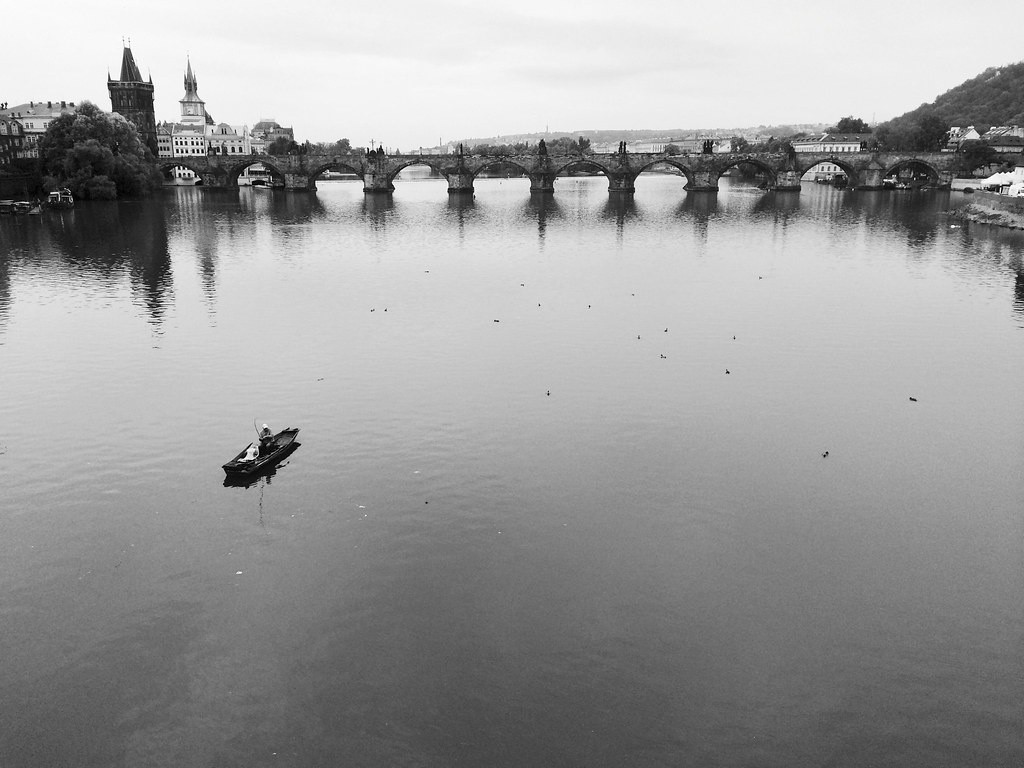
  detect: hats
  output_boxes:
[262,424,268,429]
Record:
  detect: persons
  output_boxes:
[258,423,275,454]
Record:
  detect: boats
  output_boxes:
[221,425,301,481]
[0,186,74,218]
[221,442,301,490]
[819,170,914,192]
